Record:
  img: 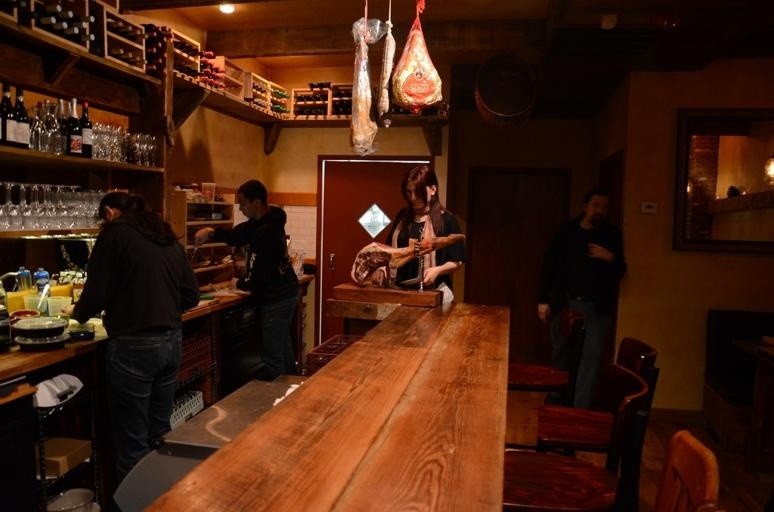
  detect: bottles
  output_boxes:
[272,88,292,112]
[294,92,324,114]
[251,79,272,109]
[38,5,96,42]
[145,22,169,76]
[0,81,93,159]
[58,271,85,304]
[15,267,32,289]
[174,39,227,88]
[331,88,351,113]
[108,19,149,65]
[33,267,50,293]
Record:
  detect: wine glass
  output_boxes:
[130,133,158,166]
[93,123,129,163]
[0,182,103,229]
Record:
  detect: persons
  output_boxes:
[534,188,628,409]
[61,190,200,482]
[192,178,300,378]
[371,163,468,303]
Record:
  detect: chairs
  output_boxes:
[653,430,719,512]
[538,336,657,476]
[504,364,647,512]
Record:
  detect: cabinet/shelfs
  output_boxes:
[0,0,169,85]
[169,188,235,271]
[169,28,448,128]
[1,130,163,310]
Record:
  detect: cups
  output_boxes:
[201,182,216,202]
[23,294,48,314]
[47,296,72,319]
[10,309,41,323]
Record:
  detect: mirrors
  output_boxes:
[672,106,774,258]
